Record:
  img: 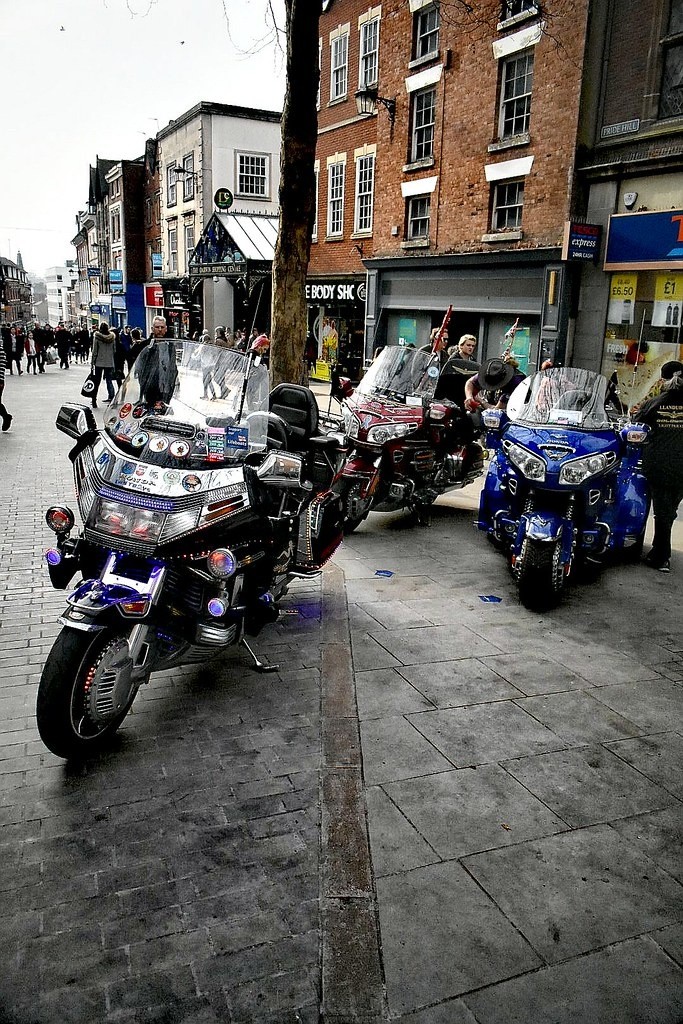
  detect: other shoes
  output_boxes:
[646,553,670,572]
[27,366,29,373]
[117,401,125,405]
[211,396,217,401]
[34,372,37,375]
[39,371,42,373]
[10,372,13,375]
[222,388,231,399]
[102,399,112,402]
[60,361,89,370]
[2,414,12,431]
[200,396,209,400]
[43,369,45,372]
[19,371,23,375]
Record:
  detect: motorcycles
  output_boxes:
[329,343,490,544]
[471,365,651,615]
[35,283,353,761]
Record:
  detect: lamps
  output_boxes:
[90,242,100,252]
[173,165,198,176]
[353,83,396,123]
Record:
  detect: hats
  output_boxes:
[662,361,683,379]
[478,358,514,390]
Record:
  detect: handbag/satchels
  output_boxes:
[81,373,99,397]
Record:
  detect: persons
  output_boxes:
[132,316,179,422]
[411,326,477,411]
[537,359,577,417]
[0,309,144,430]
[315,318,338,361]
[192,325,270,401]
[464,356,526,413]
[632,360,683,573]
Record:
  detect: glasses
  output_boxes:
[443,338,449,341]
